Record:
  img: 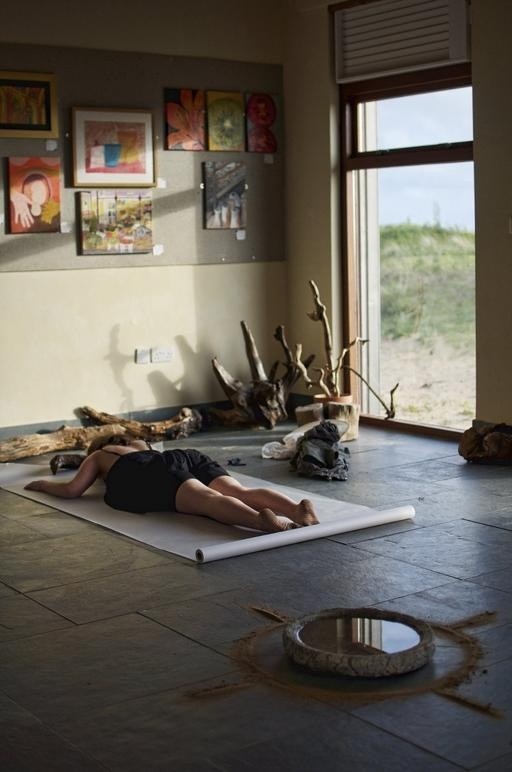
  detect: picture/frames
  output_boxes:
[70,105,158,188]
[0,69,60,139]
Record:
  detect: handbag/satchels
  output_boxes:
[288,420,351,481]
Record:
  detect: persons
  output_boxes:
[10,172,53,231]
[23,433,320,533]
